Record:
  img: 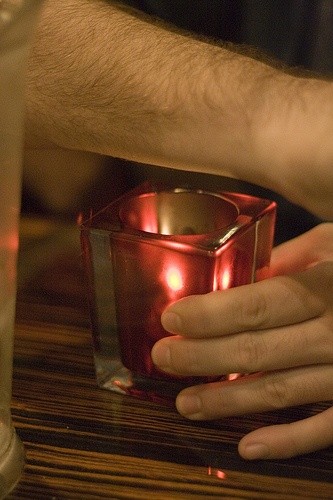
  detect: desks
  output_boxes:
[1,211,333,500]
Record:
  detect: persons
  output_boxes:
[23,0,333,462]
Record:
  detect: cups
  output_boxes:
[0,0,26,500]
[75,178,277,402]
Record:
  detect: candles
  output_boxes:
[77,174,278,407]
